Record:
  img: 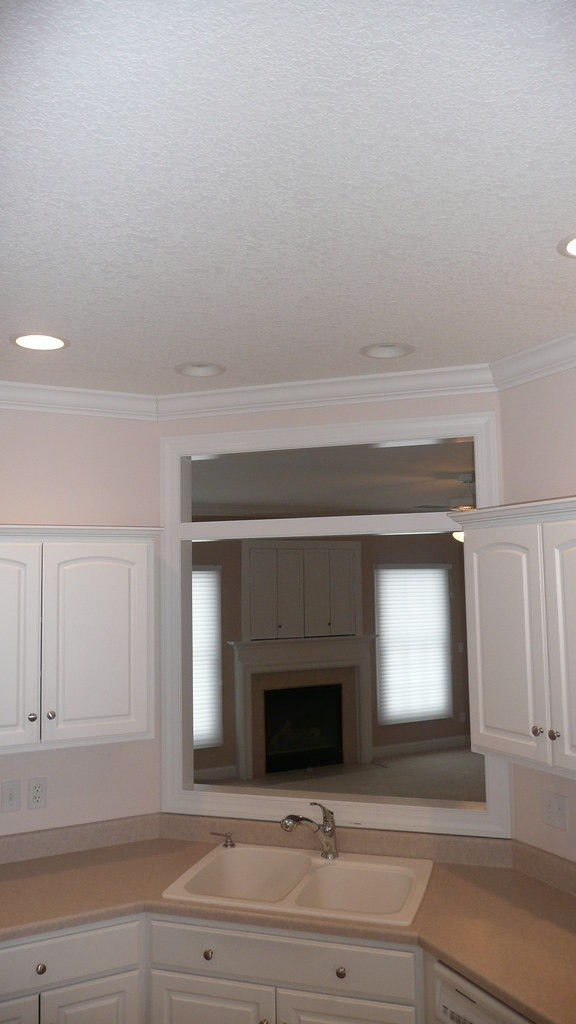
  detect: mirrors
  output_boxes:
[189,536,486,807]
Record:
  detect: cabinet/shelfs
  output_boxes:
[0,910,426,1024]
[241,540,363,639]
[1,523,155,754]
[448,498,576,779]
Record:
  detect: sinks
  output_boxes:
[161,847,312,910]
[283,858,435,926]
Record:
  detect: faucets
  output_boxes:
[280,801,339,860]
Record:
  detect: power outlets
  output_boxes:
[544,790,569,832]
[27,777,47,809]
